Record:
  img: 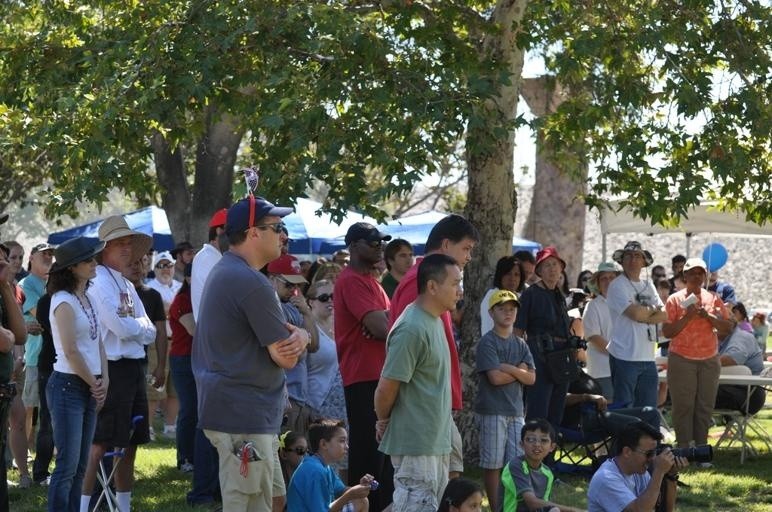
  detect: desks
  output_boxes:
[653,372,771,464]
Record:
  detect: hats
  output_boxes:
[97,216,154,265]
[612,241,654,268]
[209,208,227,227]
[534,247,565,277]
[225,196,294,233]
[267,254,310,286]
[587,262,623,297]
[683,257,708,276]
[53,235,106,271]
[170,242,198,254]
[154,252,176,267]
[345,222,391,247]
[489,289,522,310]
[31,243,57,254]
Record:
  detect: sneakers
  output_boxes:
[18,474,32,490]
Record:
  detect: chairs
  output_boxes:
[549,399,630,475]
[93,413,145,512]
[657,357,771,455]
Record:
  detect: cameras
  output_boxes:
[657,445,712,469]
[569,334,588,350]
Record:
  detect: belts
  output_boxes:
[0,382,18,399]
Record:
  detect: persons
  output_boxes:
[1,195,772,512]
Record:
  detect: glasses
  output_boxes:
[287,446,310,456]
[652,271,666,278]
[522,435,551,444]
[243,223,284,233]
[632,445,662,459]
[156,262,174,269]
[354,238,381,247]
[274,276,294,288]
[311,293,332,302]
